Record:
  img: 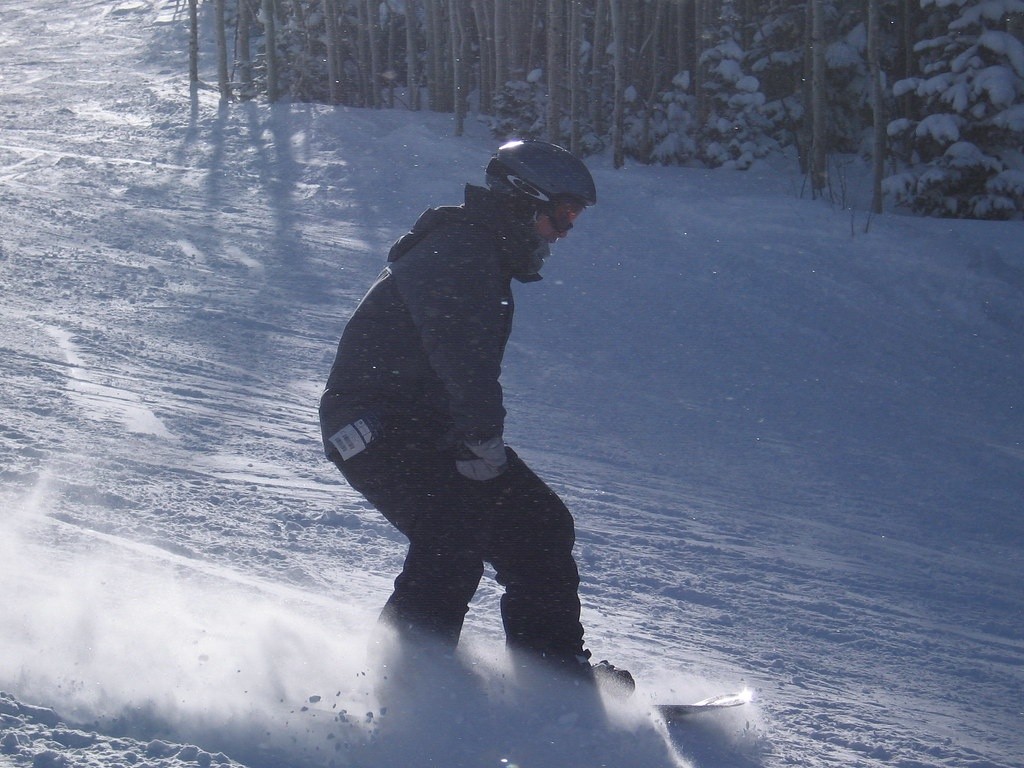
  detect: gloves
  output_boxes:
[444,431,513,482]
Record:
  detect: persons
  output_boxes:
[319,138,636,700]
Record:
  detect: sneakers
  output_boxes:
[579,661,639,705]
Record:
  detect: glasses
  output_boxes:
[551,213,574,233]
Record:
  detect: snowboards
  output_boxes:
[646,684,748,712]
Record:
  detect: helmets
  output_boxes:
[486,138,597,225]
